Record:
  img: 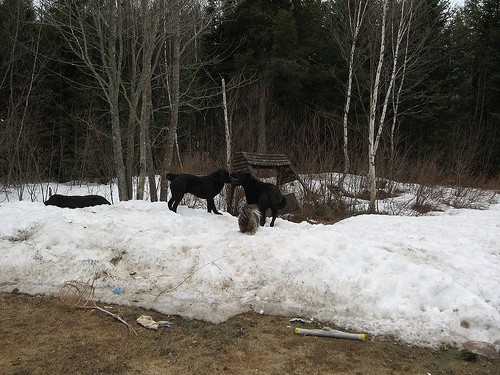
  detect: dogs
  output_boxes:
[232,172,287,227]
[166,169,232,215]
[43,194,111,209]
